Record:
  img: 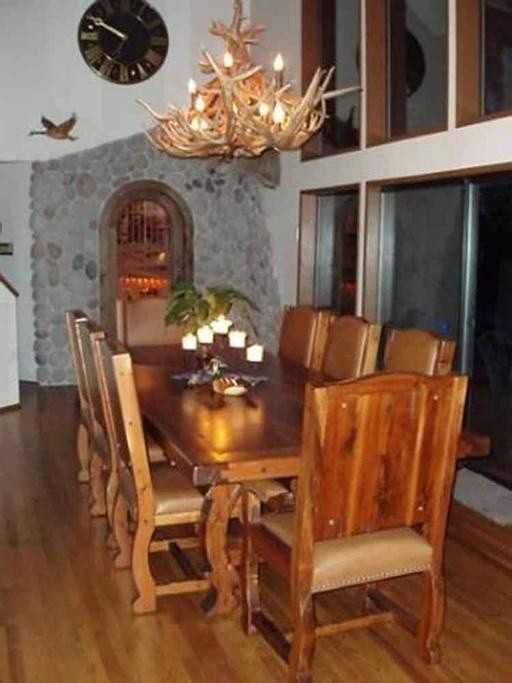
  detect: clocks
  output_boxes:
[75,0,169,85]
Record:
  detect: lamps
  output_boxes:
[133,1,361,159]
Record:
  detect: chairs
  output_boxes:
[63,293,458,615]
[240,371,469,681]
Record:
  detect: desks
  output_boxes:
[117,342,490,636]
